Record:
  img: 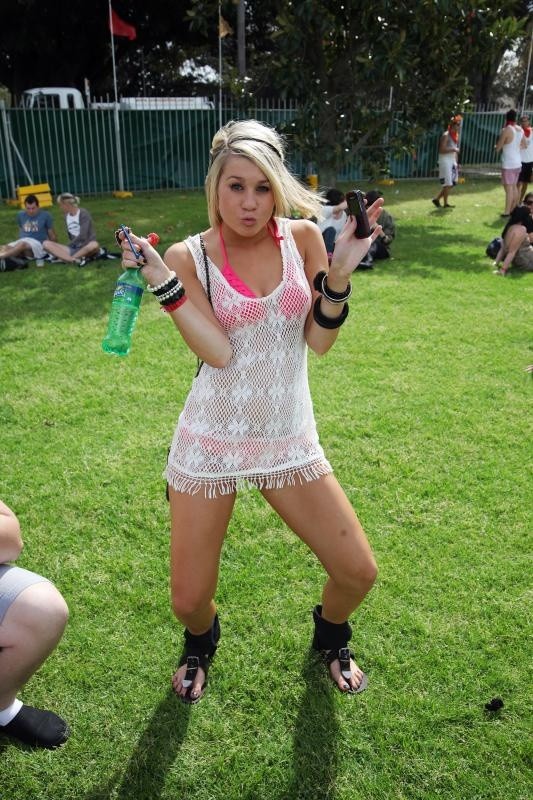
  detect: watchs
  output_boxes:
[493,261,498,266]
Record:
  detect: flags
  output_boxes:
[110,8,136,39]
[219,16,233,37]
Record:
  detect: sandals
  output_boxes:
[169,613,221,702]
[313,604,370,694]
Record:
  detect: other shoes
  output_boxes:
[444,203,455,208]
[432,199,441,207]
[1,703,69,751]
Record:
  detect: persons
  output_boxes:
[115,122,385,704]
[490,192,533,276]
[0,500,69,751]
[495,110,527,218]
[298,188,395,271]
[432,115,462,208]
[42,192,100,267]
[0,195,57,260]
[518,115,533,203]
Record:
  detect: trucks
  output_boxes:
[17,87,217,167]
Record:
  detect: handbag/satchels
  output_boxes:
[485,237,501,260]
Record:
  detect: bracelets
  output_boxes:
[313,296,353,329]
[501,268,506,272]
[312,271,354,305]
[147,270,188,313]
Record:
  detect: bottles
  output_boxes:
[101,267,147,356]
